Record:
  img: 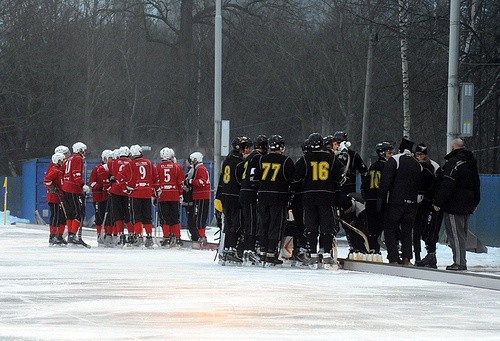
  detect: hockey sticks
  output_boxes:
[99,196,114,248]
[78,191,92,249]
[154,199,161,249]
[337,216,370,254]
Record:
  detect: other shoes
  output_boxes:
[218,247,338,269]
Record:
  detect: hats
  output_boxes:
[340,198,352,211]
[415,143,427,155]
[398,138,415,151]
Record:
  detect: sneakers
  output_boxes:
[390,253,467,271]
[49,231,207,250]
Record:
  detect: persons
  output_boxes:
[438,138,481,270]
[214,132,443,269]
[41,142,211,248]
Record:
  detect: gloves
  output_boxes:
[155,187,162,199]
[99,185,103,191]
[107,172,116,183]
[340,141,348,152]
[82,185,90,194]
[50,185,58,193]
[152,197,157,207]
[184,178,193,187]
[179,194,183,207]
[122,186,133,195]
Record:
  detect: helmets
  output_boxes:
[54,145,69,154]
[52,153,65,166]
[72,142,87,153]
[232,131,349,153]
[101,143,204,164]
[376,142,395,161]
